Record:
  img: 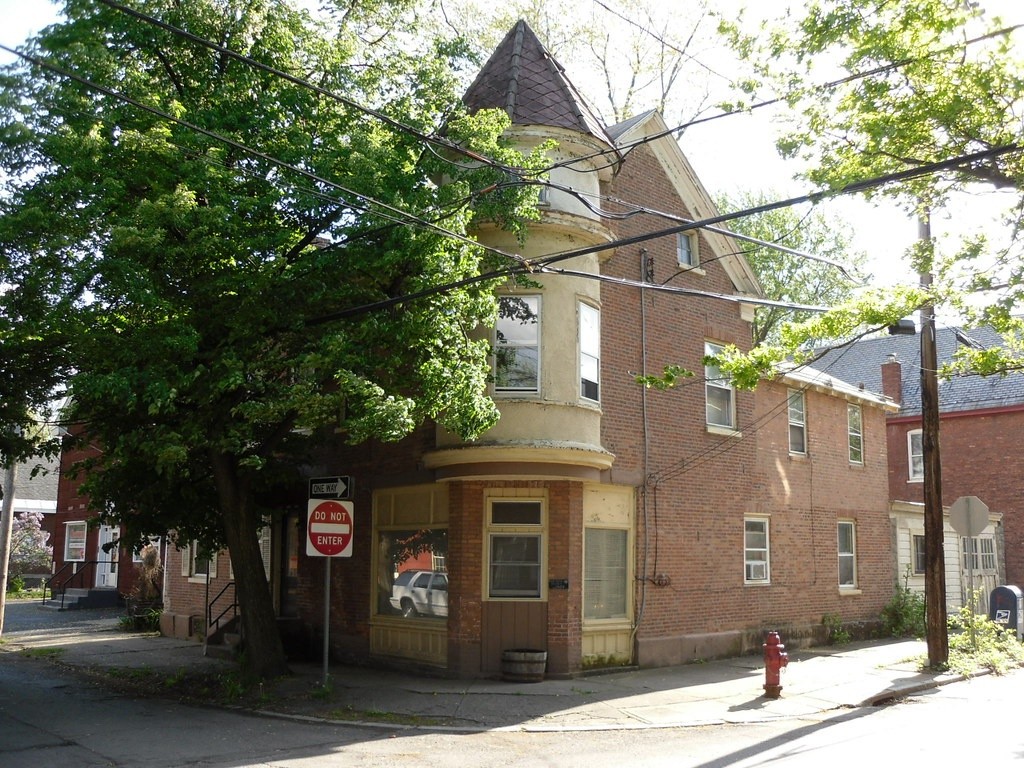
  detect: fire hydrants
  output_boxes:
[762,630,788,697]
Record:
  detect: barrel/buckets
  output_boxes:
[502,649,547,682]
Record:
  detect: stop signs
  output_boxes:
[306,499,354,557]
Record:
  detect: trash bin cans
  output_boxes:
[989,585,1024,642]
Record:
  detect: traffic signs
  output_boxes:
[309,476,351,500]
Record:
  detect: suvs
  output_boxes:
[391,568,450,618]
